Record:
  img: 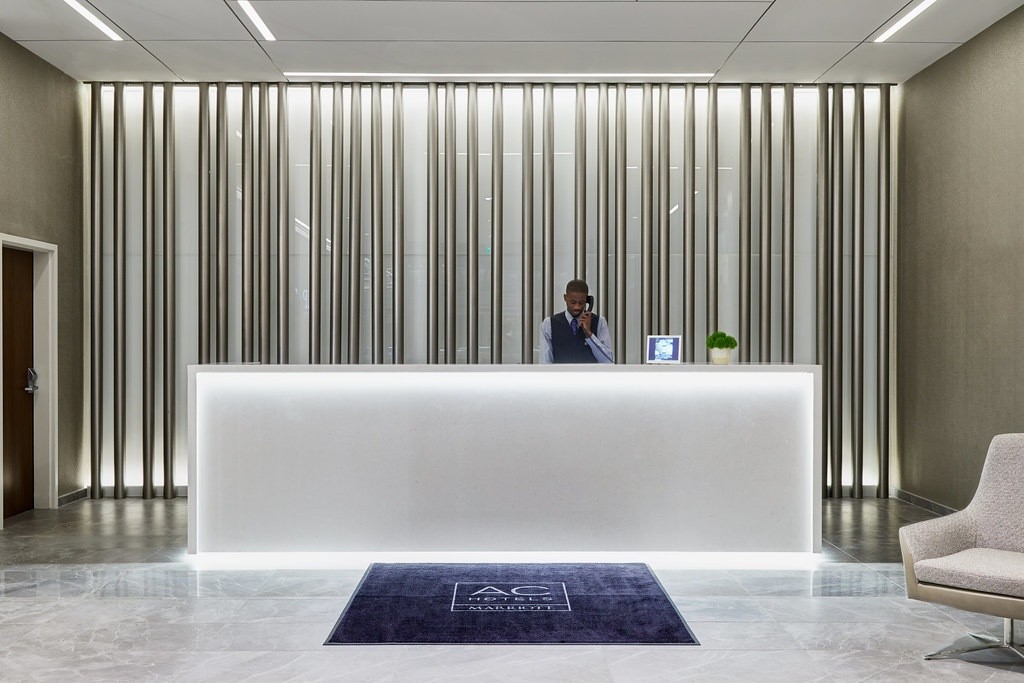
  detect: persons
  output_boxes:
[538,279,613,363]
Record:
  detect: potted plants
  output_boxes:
[706,331,737,364]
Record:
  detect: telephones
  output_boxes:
[586,296,594,312]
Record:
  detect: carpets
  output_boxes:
[322,562,701,645]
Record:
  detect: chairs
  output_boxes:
[899,434,1024,666]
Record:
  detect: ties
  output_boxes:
[570,319,579,336]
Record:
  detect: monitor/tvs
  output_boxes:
[646,335,681,364]
